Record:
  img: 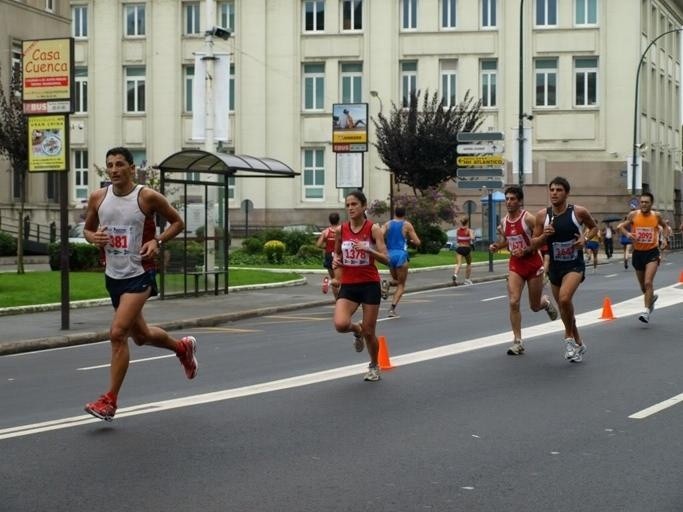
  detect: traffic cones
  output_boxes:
[679,272,683,283]
[598,297,617,321]
[377,334,394,371]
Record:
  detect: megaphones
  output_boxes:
[636,142,646,150]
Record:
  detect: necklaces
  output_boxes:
[550,203,567,225]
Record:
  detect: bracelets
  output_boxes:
[522,247,528,255]
[154,237,161,247]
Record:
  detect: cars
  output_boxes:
[279,222,323,240]
[443,228,483,248]
[51,221,92,244]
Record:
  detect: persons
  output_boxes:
[316,213,339,301]
[330,190,392,380]
[583,192,672,323]
[82,146,200,421]
[488,186,557,355]
[379,205,421,317]
[451,216,475,285]
[342,110,365,130]
[529,177,598,361]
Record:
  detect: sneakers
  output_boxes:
[647,294,658,313]
[506,341,526,356]
[363,363,383,382]
[176,336,199,380]
[463,278,473,285]
[352,319,365,352]
[571,338,588,363]
[452,273,458,287]
[543,294,558,321]
[386,308,399,318]
[381,279,390,301]
[638,307,651,323]
[562,334,577,361]
[84,391,118,423]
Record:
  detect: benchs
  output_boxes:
[186,271,227,298]
